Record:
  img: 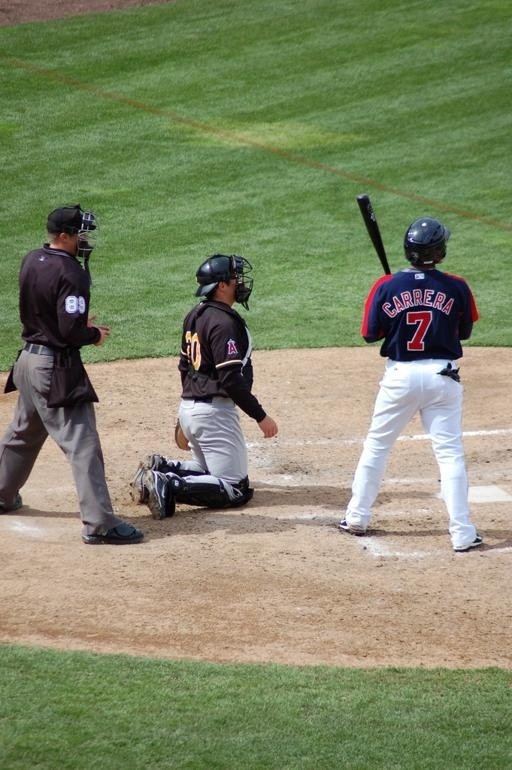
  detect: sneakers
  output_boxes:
[338,519,366,534]
[0,495,22,514]
[130,455,185,519]
[82,521,144,544]
[453,533,482,552]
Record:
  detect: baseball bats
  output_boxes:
[355,192,392,277]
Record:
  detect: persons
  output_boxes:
[0,203,144,546]
[334,216,485,552]
[129,252,279,520]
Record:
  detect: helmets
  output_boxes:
[48,204,96,257]
[404,218,451,265]
[196,255,253,311]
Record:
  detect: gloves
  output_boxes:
[439,363,459,382]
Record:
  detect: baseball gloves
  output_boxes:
[175,418,191,453]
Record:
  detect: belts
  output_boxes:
[24,343,53,356]
